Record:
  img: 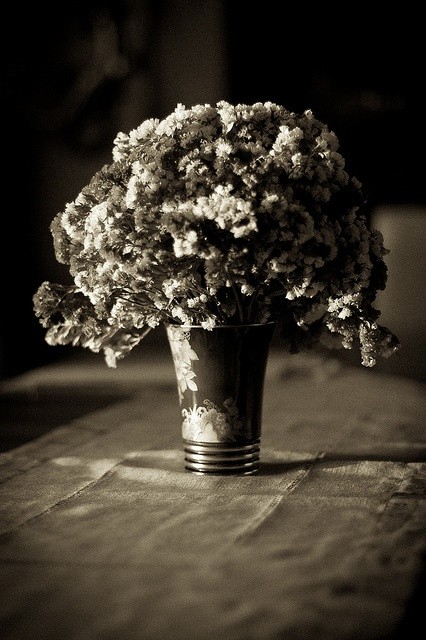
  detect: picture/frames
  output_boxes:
[165,322,273,474]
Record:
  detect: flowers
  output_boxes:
[32,101,400,369]
